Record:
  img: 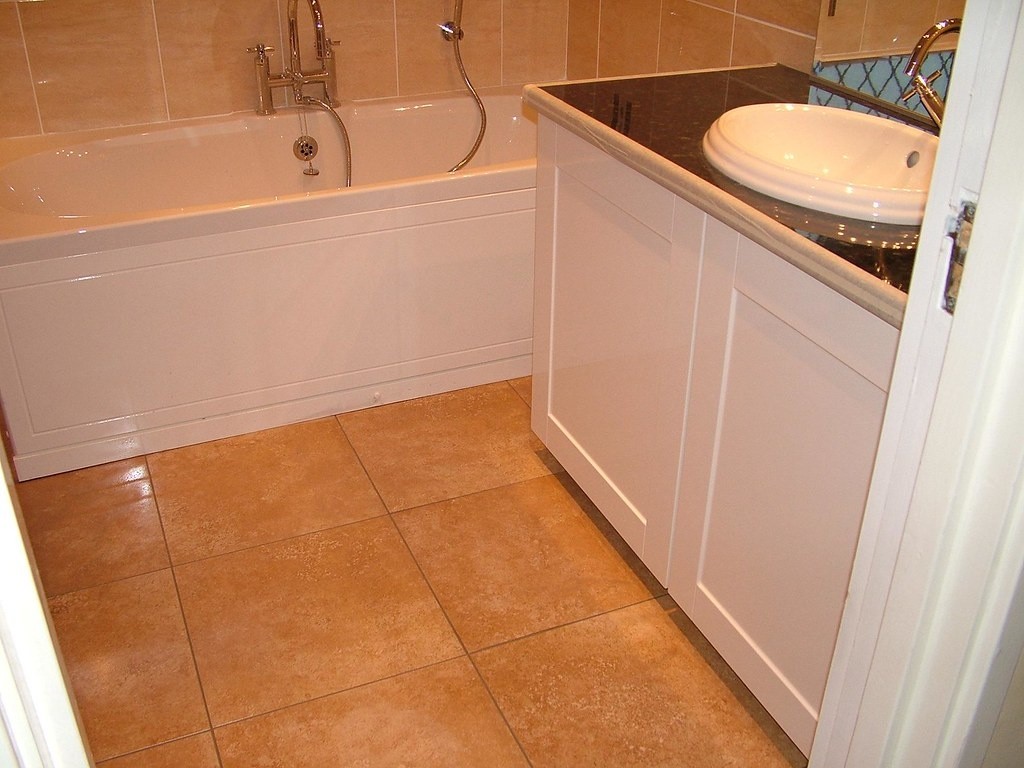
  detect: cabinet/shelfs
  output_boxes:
[532,112,902,761]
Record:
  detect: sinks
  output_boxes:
[702,103,939,225]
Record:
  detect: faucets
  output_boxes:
[283,0,329,106]
[903,18,961,78]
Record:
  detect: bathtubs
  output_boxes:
[1,92,538,482]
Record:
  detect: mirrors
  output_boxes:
[812,1,969,127]
